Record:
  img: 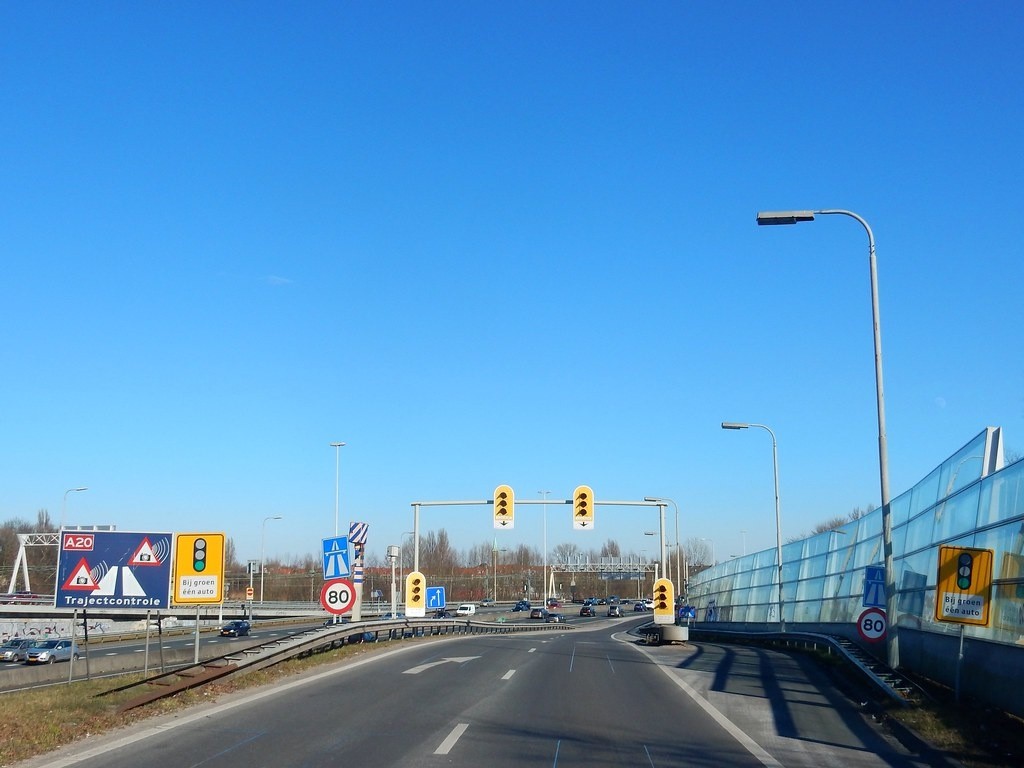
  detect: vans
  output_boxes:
[455,603,476,617]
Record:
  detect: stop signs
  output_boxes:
[246,587,254,600]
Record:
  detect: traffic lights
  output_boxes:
[405,571,426,618]
[494,484,515,530]
[573,485,595,531]
[652,578,675,625]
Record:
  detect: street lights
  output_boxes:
[537,489,553,611]
[665,542,686,599]
[60,486,89,530]
[330,442,345,536]
[701,537,716,567]
[644,531,672,582]
[260,515,283,604]
[644,497,681,598]
[756,207,901,671]
[399,531,415,604]
[721,421,786,634]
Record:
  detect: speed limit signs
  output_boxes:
[857,608,887,644]
[320,578,356,615]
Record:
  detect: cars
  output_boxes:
[580,594,654,617]
[2,591,43,606]
[23,639,80,664]
[377,611,405,620]
[0,638,40,663]
[543,598,562,608]
[530,607,549,619]
[479,598,496,607]
[433,611,451,618]
[512,600,531,612]
[545,613,566,623]
[605,605,626,617]
[580,606,597,617]
[220,621,252,638]
